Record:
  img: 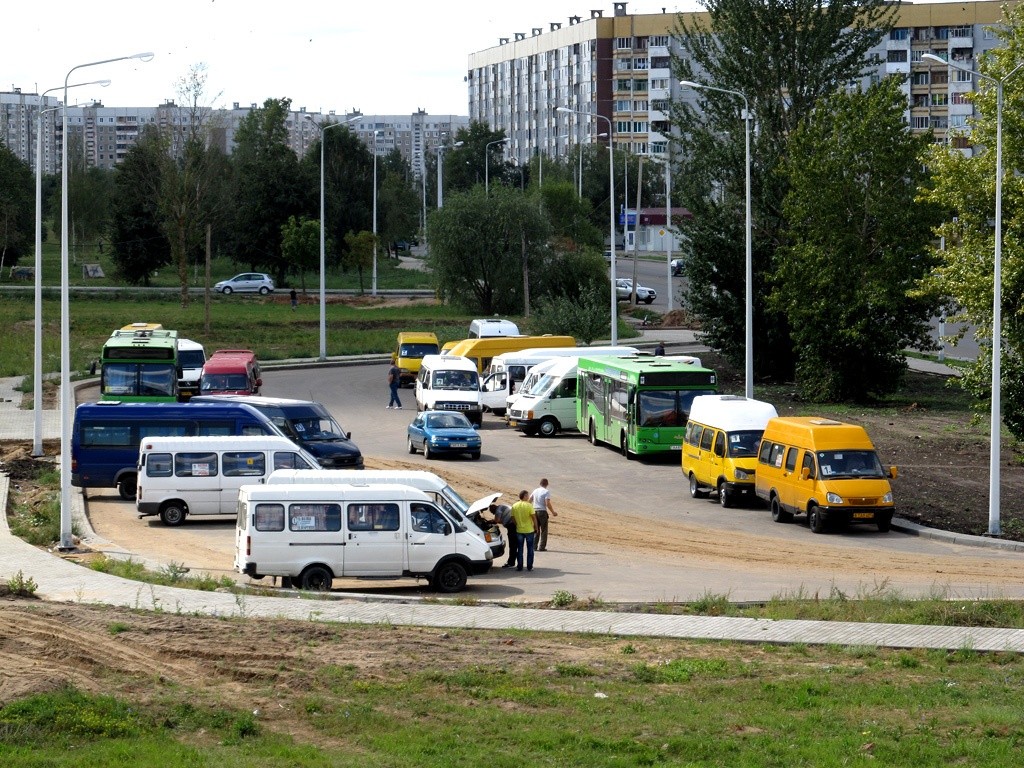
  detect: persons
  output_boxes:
[753,432,762,452]
[845,451,865,471]
[528,478,558,551]
[655,341,665,356]
[458,373,472,386]
[484,365,491,380]
[490,504,518,568]
[290,287,300,312]
[411,504,421,531]
[500,372,516,395]
[511,490,539,572]
[386,360,403,409]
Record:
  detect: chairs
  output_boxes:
[322,504,413,533]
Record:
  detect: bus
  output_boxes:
[120,323,164,331]
[577,354,718,460]
[89,330,183,403]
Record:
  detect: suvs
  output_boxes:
[602,252,617,266]
[615,278,656,304]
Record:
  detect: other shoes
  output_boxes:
[518,567,523,571]
[501,562,514,568]
[394,406,402,410]
[539,548,547,551]
[386,405,393,409]
[533,548,537,551]
[528,567,533,571]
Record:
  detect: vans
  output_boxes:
[73,401,291,498]
[478,347,640,388]
[505,358,559,425]
[234,484,493,591]
[199,348,261,395]
[177,338,205,398]
[393,316,577,380]
[682,394,779,507]
[267,469,507,558]
[755,417,897,533]
[190,394,365,471]
[136,435,325,525]
[511,357,578,438]
[417,355,509,428]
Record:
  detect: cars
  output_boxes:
[407,410,482,459]
[670,258,694,276]
[215,272,274,296]
